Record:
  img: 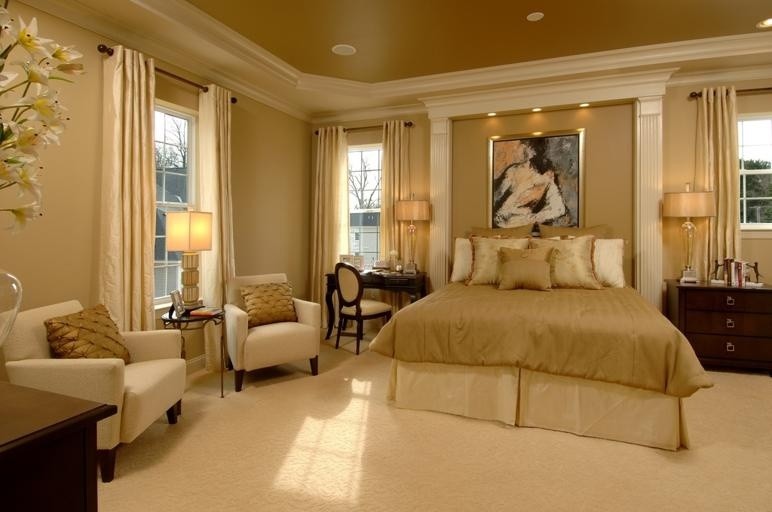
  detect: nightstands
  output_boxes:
[664,279,772,376]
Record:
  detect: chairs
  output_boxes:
[1,300,189,483]
[224,273,321,393]
[334,262,392,355]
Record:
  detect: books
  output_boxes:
[723,258,750,287]
[189,306,224,317]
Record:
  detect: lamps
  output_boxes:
[395,190,430,276]
[165,211,213,312]
[663,182,718,283]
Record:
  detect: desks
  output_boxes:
[162,305,226,410]
[324,267,427,341]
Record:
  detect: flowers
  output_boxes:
[0,8,86,235]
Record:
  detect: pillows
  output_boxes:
[526,233,607,291]
[497,245,560,293]
[568,234,627,289]
[466,234,530,287]
[239,279,298,329]
[449,236,473,283]
[43,303,131,364]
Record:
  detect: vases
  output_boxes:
[0,266,33,352]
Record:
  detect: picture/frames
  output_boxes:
[169,288,186,318]
[339,254,355,267]
[354,256,365,272]
[487,127,586,233]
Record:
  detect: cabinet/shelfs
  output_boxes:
[0,382,118,512]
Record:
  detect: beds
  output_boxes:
[367,224,714,453]
